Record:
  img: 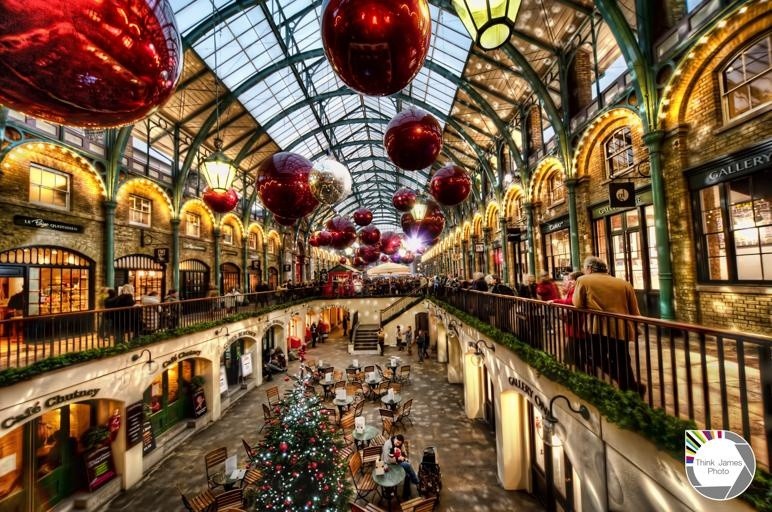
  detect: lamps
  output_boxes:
[196,1,239,194]
[534,395,589,447]
[132,348,158,375]
[451,0,524,53]
[470,338,495,367]
[408,169,428,223]
[445,320,462,338]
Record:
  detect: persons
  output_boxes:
[343,314,348,337]
[394,325,431,364]
[334,274,423,299]
[224,286,236,316]
[254,275,327,313]
[426,273,595,379]
[390,447,408,462]
[380,433,421,501]
[196,394,205,410]
[233,283,241,314]
[47,429,78,472]
[262,346,288,374]
[310,321,318,348]
[102,275,184,341]
[317,319,328,343]
[206,284,220,317]
[573,256,648,401]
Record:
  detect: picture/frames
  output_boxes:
[124,399,144,451]
